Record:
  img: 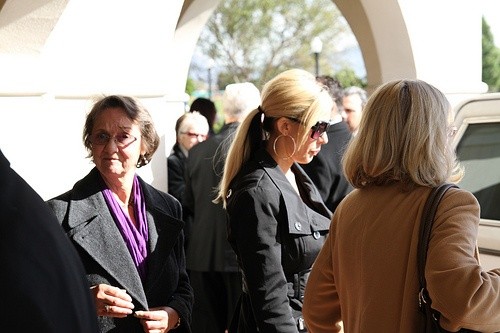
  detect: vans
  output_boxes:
[443,91,500,278]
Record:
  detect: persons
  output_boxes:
[0,148,99,333]
[300,75,354,211]
[167,110,208,205]
[183,82,257,333]
[190,98,217,128]
[302,78,500,333]
[341,87,367,131]
[45,94,194,333]
[213,70,333,333]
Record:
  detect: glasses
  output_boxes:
[344,108,364,115]
[282,115,331,140]
[181,128,207,142]
[89,131,144,146]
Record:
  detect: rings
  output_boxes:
[104,305,108,312]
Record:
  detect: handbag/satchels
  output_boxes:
[417,184,482,333]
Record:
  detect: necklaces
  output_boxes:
[120,196,133,207]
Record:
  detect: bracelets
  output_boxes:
[174,317,180,328]
